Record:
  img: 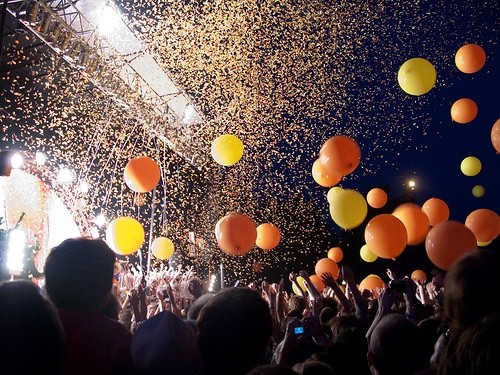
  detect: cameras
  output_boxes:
[293,326,304,335]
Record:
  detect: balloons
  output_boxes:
[309,118,500,298]
[397,57,437,96]
[450,98,478,123]
[105,216,144,256]
[292,276,307,296]
[124,156,160,193]
[151,236,174,260]
[255,223,280,250]
[214,214,257,256]
[455,44,486,74]
[211,133,244,167]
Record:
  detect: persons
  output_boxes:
[43,238,136,375]
[103,254,499,375]
[0,280,65,375]
